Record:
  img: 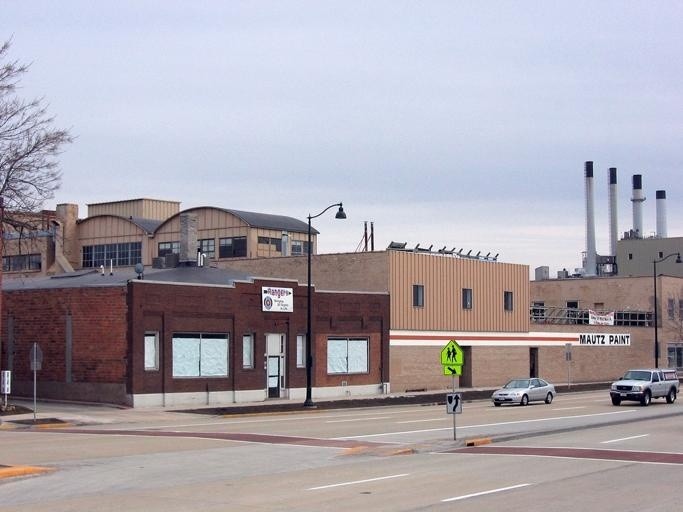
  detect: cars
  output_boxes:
[491,378,557,406]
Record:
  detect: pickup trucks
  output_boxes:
[609,368,680,406]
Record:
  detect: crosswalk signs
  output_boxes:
[440,339,465,376]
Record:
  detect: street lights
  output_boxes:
[653,252,683,368]
[304,201,347,409]
